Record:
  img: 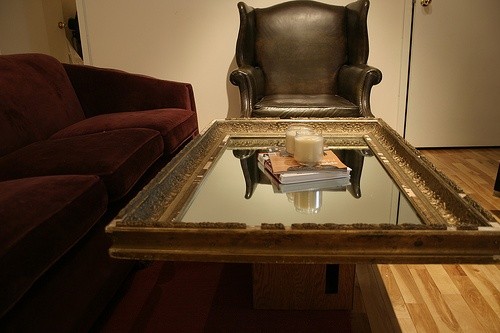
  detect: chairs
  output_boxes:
[230,1,383,119]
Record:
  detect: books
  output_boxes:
[258,163,352,192]
[257,147,351,185]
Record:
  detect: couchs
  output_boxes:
[0,53,200,332]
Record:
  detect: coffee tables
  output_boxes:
[102,117,500,309]
